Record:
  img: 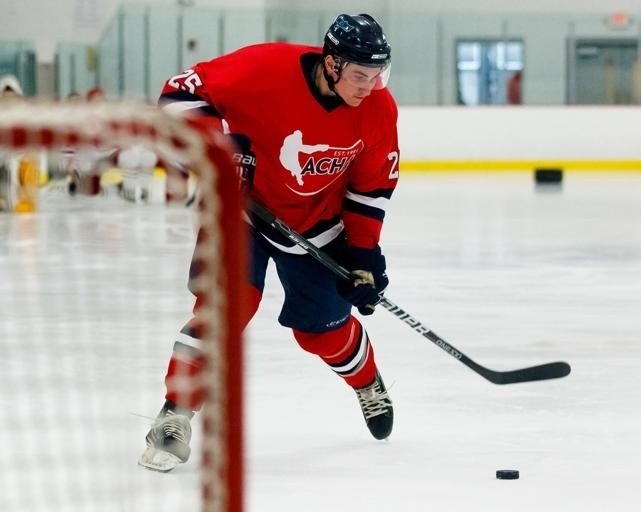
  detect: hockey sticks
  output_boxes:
[245,198,570,385]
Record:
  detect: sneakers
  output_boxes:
[353,370,392,439]
[146,400,194,463]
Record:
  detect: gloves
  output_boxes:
[336,245,388,315]
[227,134,256,192]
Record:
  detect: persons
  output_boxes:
[0,71,205,217]
[141,12,404,465]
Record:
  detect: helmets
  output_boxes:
[325,14,392,90]
